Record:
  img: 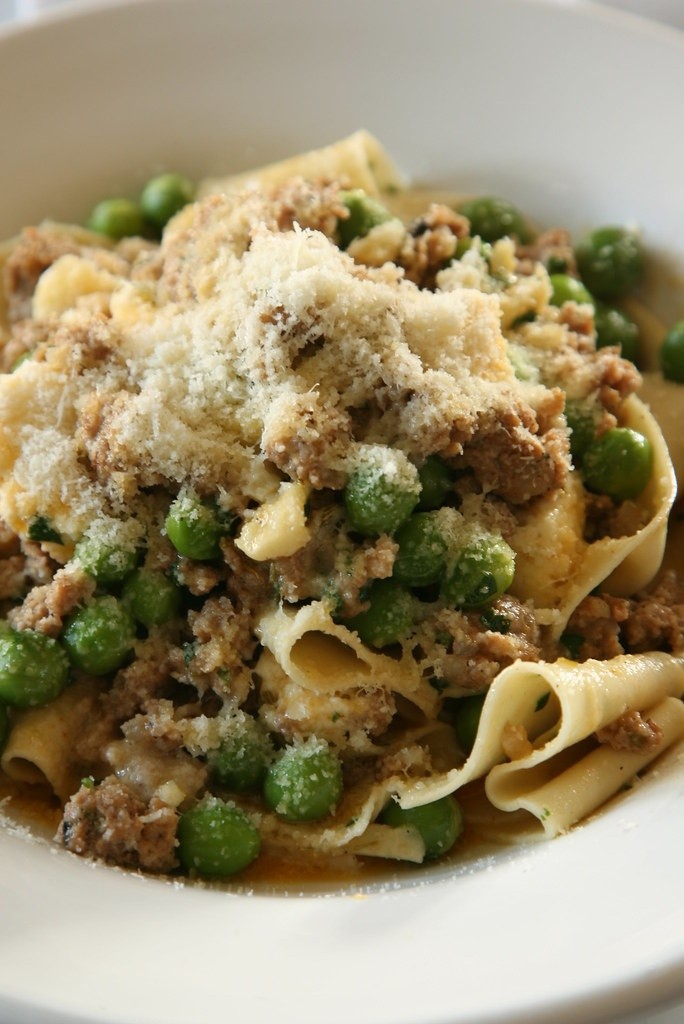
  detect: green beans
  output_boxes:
[0,172,684,880]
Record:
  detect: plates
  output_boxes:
[0,0,684,1024]
